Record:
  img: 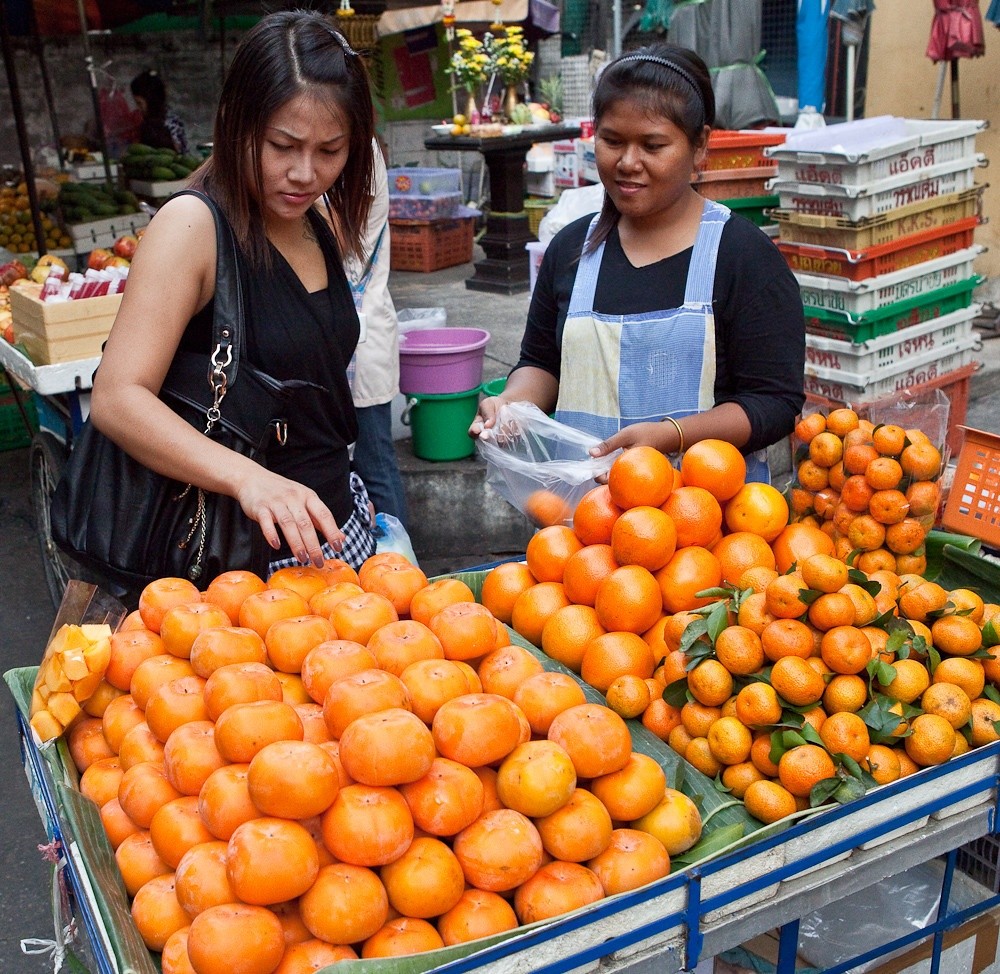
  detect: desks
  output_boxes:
[428,122,589,300]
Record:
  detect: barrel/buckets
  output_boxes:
[399,383,484,462]
[483,376,556,420]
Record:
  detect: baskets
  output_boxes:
[576,111,981,402]
[800,361,975,459]
[940,421,1000,552]
[939,834,999,900]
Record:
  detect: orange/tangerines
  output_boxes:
[1,78,999,974]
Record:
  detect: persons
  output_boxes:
[127,66,190,160]
[465,40,807,486]
[311,131,412,536]
[82,12,380,584]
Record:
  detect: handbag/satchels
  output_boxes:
[371,511,420,571]
[478,404,625,531]
[47,189,326,592]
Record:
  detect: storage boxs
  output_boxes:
[391,192,465,218]
[387,165,460,196]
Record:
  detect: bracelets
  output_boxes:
[661,416,684,459]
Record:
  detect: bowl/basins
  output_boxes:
[399,328,490,394]
